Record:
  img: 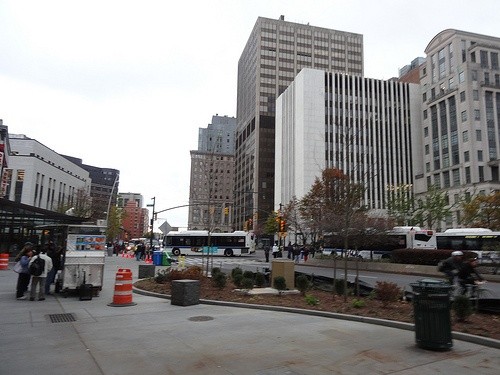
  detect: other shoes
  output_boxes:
[30,297,35,301]
[17,296,27,300]
[39,298,45,300]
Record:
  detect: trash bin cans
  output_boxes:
[153,251,162,266]
[162,251,171,265]
[408,278,454,352]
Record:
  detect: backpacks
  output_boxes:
[29,254,45,276]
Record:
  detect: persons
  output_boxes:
[113,239,147,262]
[14,242,64,296]
[263,241,315,263]
[458,256,487,299]
[436,251,464,285]
[27,245,53,302]
[15,248,34,300]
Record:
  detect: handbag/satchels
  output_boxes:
[13,261,23,273]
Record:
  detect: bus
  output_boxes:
[321,226,438,261]
[162,230,257,257]
[435,228,500,267]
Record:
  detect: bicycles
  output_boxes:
[408,279,500,311]
